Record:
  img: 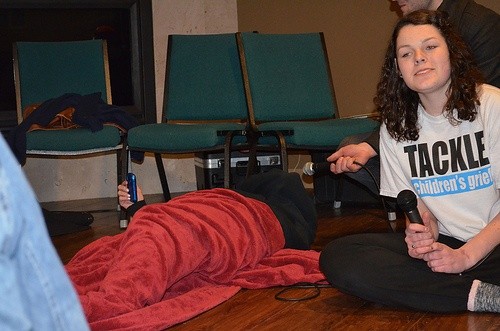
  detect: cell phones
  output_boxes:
[125,173,137,202]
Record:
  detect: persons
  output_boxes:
[319,9,500,313]
[117,167,316,250]
[0,132,91,331]
[326,0,500,206]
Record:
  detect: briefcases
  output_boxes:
[195,150,281,191]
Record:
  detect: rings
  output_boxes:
[412,244,416,249]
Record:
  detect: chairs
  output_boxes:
[12,37,127,213]
[119,29,259,229]
[234,30,381,180]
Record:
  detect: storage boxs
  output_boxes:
[193,151,284,191]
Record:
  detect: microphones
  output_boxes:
[396,190,424,233]
[302,159,336,176]
